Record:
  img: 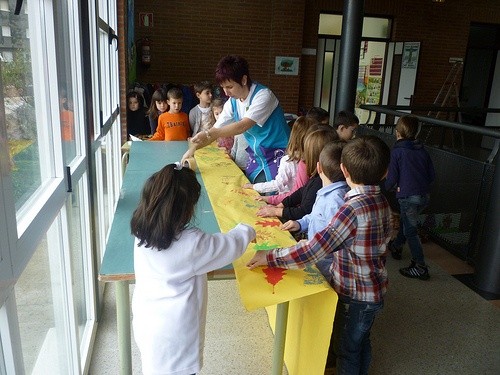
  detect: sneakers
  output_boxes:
[388,240,402,260]
[399,259,430,280]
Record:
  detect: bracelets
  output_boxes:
[204,129,211,140]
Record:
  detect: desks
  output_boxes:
[98,141,339,375]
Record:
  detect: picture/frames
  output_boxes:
[274,56,299,76]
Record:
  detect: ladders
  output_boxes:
[412,62,466,149]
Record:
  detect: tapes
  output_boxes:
[182,158,197,172]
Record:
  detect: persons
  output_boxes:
[246,134,395,375]
[59,85,75,141]
[1,81,37,142]
[384,115,436,281]
[130,162,257,375]
[127,53,360,285]
